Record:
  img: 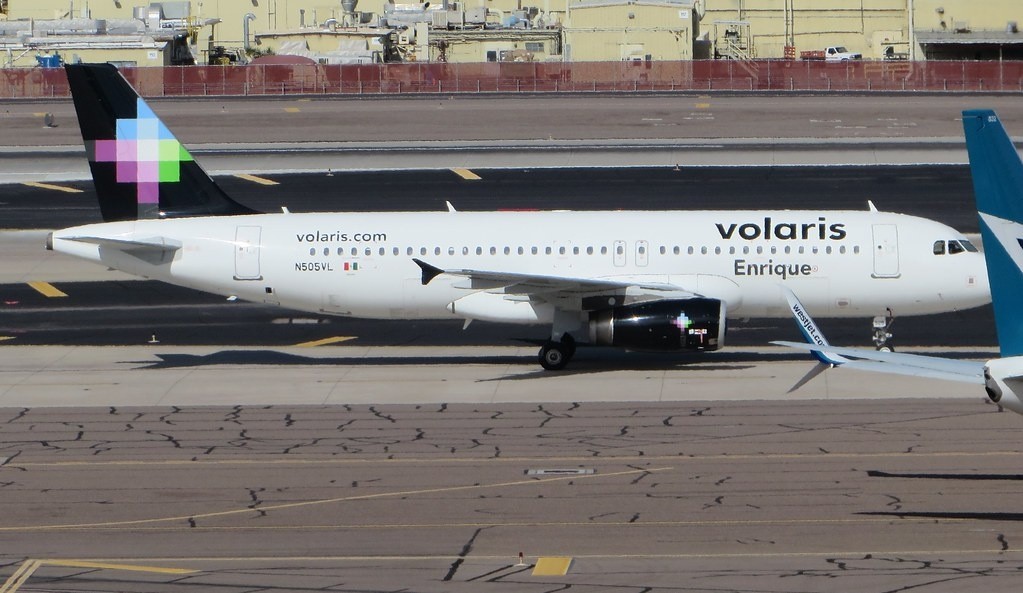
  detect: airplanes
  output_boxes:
[768,106,1022,416]
[45,62,993,370]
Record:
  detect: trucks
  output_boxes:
[798,47,863,69]
